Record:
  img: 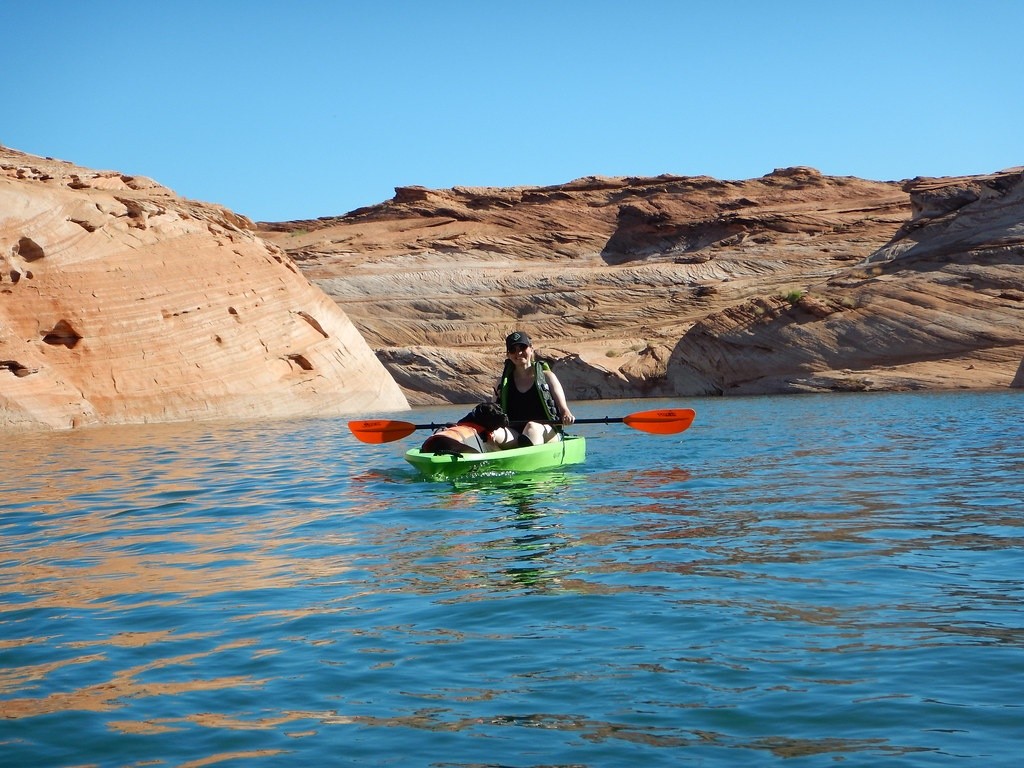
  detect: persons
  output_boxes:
[483,332,575,449]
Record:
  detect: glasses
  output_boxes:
[506,345,528,354]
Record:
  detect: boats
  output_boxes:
[404,434,587,483]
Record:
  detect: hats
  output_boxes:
[506,331,531,347]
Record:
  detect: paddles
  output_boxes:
[348,408,696,444]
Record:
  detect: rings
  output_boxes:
[564,415,567,417]
